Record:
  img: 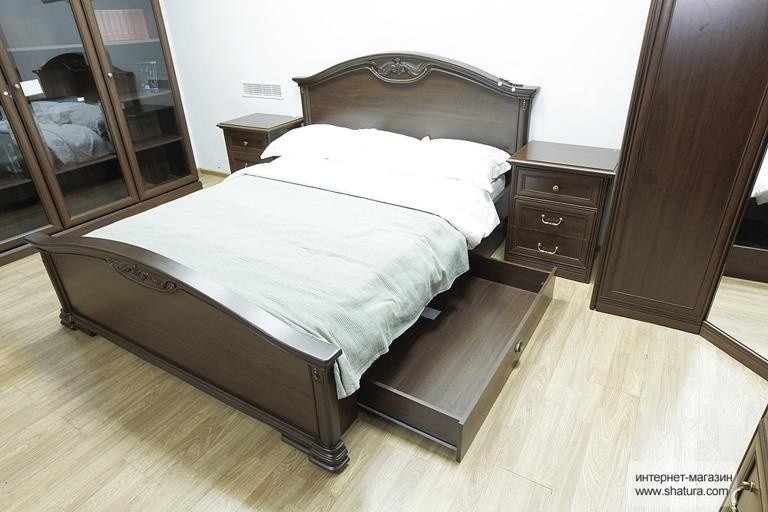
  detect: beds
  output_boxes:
[20,52,557,476]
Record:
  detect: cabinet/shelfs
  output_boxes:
[0,1,204,267]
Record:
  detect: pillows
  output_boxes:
[260,123,512,194]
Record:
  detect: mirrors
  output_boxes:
[706,144,767,364]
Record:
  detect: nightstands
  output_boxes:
[503,140,622,284]
[215,113,304,175]
